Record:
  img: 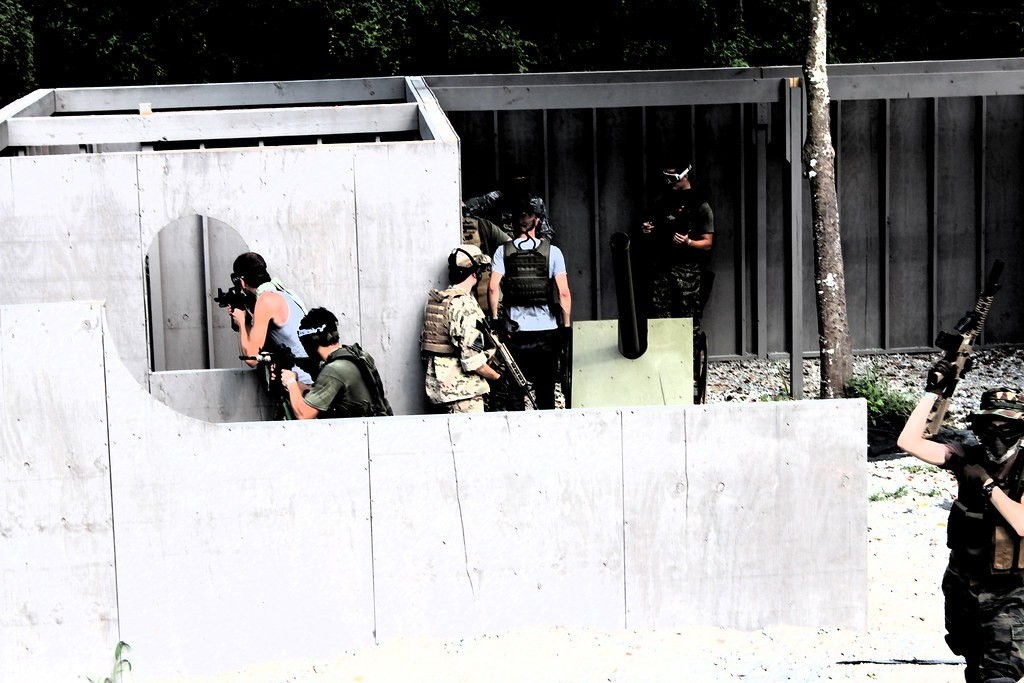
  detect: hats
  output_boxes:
[970,387,1024,420]
[451,244,491,268]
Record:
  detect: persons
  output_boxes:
[462,200,512,243]
[269,307,394,419]
[896,363,1023,683]
[227,253,313,414]
[421,246,533,413]
[490,190,574,410]
[642,150,715,403]
[476,255,497,310]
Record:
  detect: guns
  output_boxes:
[214,286,257,332]
[923,260,1005,435]
[483,318,539,411]
[239,343,317,382]
[552,303,572,410]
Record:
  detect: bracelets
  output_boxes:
[688,239,692,246]
[285,378,297,385]
[982,480,998,500]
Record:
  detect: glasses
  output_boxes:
[977,422,1020,446]
[662,163,693,185]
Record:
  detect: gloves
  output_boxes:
[926,361,953,395]
[963,464,994,495]
